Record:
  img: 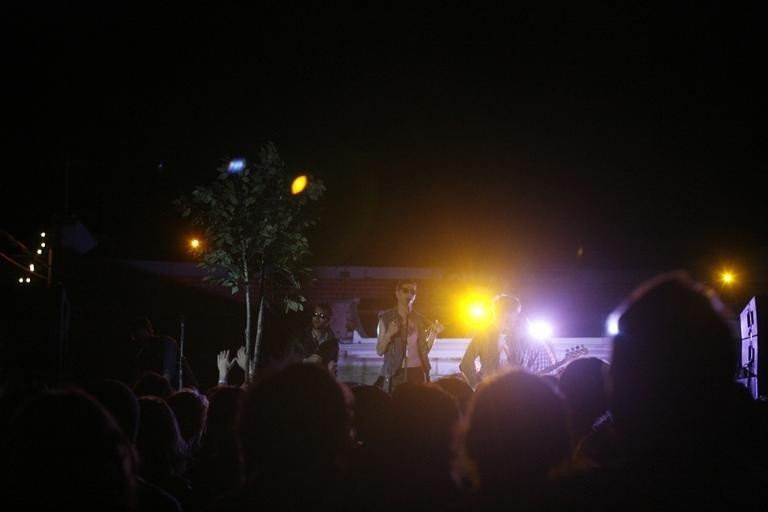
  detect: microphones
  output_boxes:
[407,298,413,312]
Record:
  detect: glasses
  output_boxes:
[312,311,330,320]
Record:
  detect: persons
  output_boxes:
[303,301,338,376]
[377,277,442,388]
[0,346,616,512]
[130,315,199,389]
[460,294,556,386]
[611,278,768,511]
